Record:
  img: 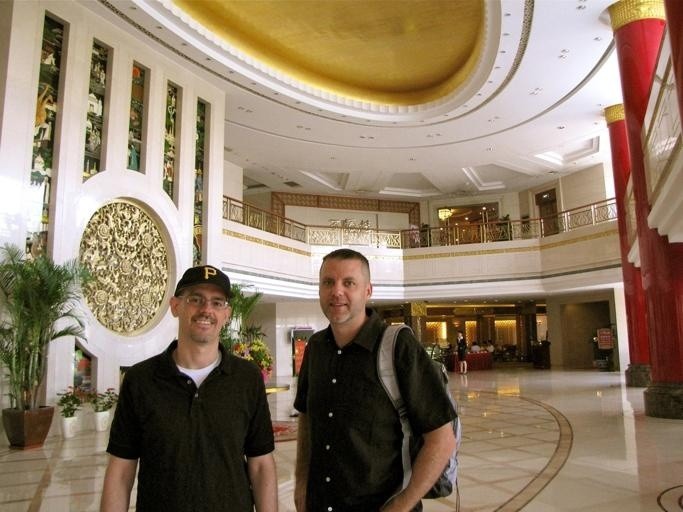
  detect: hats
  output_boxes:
[174,265,235,300]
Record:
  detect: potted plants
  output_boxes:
[1,241,95,451]
[591,327,609,370]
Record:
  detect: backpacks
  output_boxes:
[306,325,463,501]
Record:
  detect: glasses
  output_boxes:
[177,295,231,310]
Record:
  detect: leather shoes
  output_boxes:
[458,372,469,375]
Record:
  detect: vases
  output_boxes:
[60,416,79,439]
[93,410,110,433]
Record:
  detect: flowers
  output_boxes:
[228,338,275,386]
[56,382,121,416]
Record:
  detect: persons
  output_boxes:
[470,338,496,355]
[100,264,280,512]
[293,249,458,512]
[456,331,468,374]
